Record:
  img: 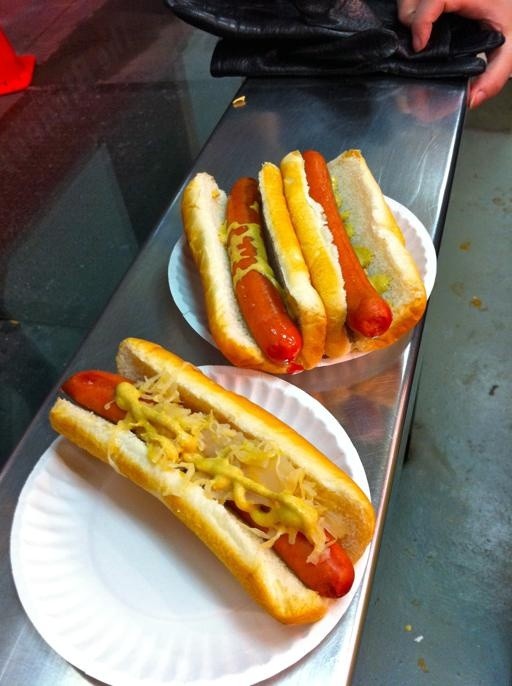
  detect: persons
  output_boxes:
[390,0,512,113]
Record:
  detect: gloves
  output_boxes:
[166,0,505,79]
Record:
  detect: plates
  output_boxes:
[167,188,439,370]
[8,364,375,686]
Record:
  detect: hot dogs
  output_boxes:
[47,335,377,628]
[279,146,428,358]
[182,161,329,377]
